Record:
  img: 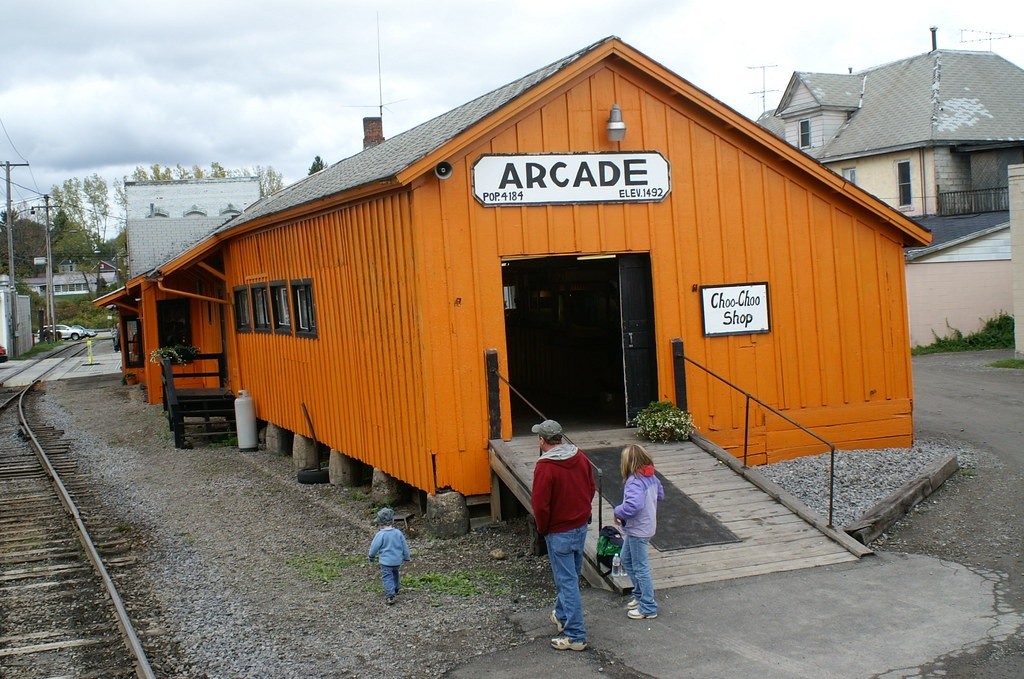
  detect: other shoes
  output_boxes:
[387,597,395,603]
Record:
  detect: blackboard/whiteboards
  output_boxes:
[699,281,772,338]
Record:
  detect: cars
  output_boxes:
[72,325,100,337]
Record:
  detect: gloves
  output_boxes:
[370,557,375,563]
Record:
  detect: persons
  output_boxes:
[368,508,410,603]
[111,323,122,353]
[613,444,665,620]
[532,420,595,650]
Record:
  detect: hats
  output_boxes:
[531,420,563,441]
[377,507,394,525]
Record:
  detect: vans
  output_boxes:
[37,324,85,341]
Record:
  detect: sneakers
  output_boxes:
[627,598,641,609]
[551,635,587,652]
[627,608,658,619]
[550,610,564,632]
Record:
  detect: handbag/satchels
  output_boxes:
[596,526,625,577]
